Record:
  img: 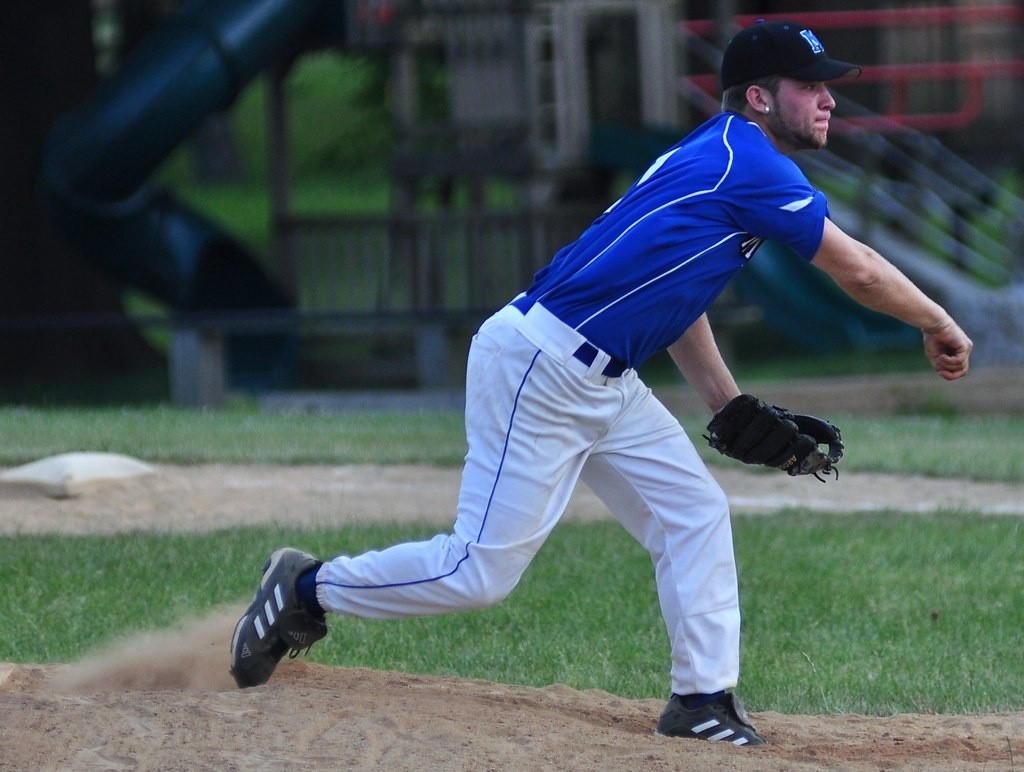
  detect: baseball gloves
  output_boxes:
[705,393,846,477]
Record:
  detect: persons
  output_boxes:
[230,16,973,745]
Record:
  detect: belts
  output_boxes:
[510,296,627,378]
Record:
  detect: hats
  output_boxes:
[721,18,862,82]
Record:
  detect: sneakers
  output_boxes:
[229,548,328,688]
[656,691,768,747]
